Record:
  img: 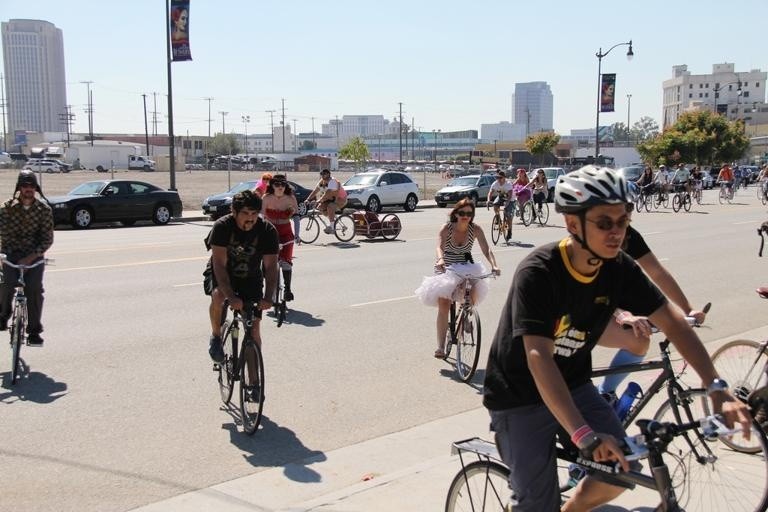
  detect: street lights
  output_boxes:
[714,79,743,114]
[595,39,633,158]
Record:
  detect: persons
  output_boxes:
[305,168,348,234]
[484,164,752,512]
[0,171,54,343]
[591,226,707,416]
[202,192,279,363]
[634,160,768,203]
[485,169,548,241]
[260,175,300,301]
[601,82,614,106]
[170,6,189,44]
[416,197,500,359]
[254,173,273,219]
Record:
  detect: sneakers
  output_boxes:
[325,227,335,233]
[28,335,43,344]
[507,233,511,239]
[435,348,445,357]
[463,320,473,332]
[600,390,620,408]
[209,336,224,363]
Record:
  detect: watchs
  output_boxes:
[704,377,728,396]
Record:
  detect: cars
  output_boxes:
[23,158,72,174]
[436,174,512,208]
[368,163,508,178]
[202,179,316,221]
[513,168,568,203]
[48,180,185,230]
[618,165,761,193]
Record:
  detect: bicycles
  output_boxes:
[635,174,767,213]
[445,405,759,511]
[439,265,499,383]
[212,293,288,435]
[521,188,550,228]
[699,285,768,452]
[556,301,768,510]
[273,240,302,327]
[0,253,57,385]
[298,200,356,244]
[486,200,515,245]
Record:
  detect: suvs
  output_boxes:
[338,171,419,211]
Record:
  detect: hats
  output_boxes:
[270,175,289,183]
[17,170,38,191]
[496,172,505,178]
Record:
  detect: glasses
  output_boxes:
[456,211,473,217]
[587,215,628,230]
[22,183,35,188]
[275,183,285,187]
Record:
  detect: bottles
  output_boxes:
[611,382,644,423]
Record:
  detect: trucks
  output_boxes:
[78,142,157,172]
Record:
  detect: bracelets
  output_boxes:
[568,421,595,446]
[436,257,443,260]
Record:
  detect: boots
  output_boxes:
[283,270,293,300]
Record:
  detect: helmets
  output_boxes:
[555,164,634,214]
